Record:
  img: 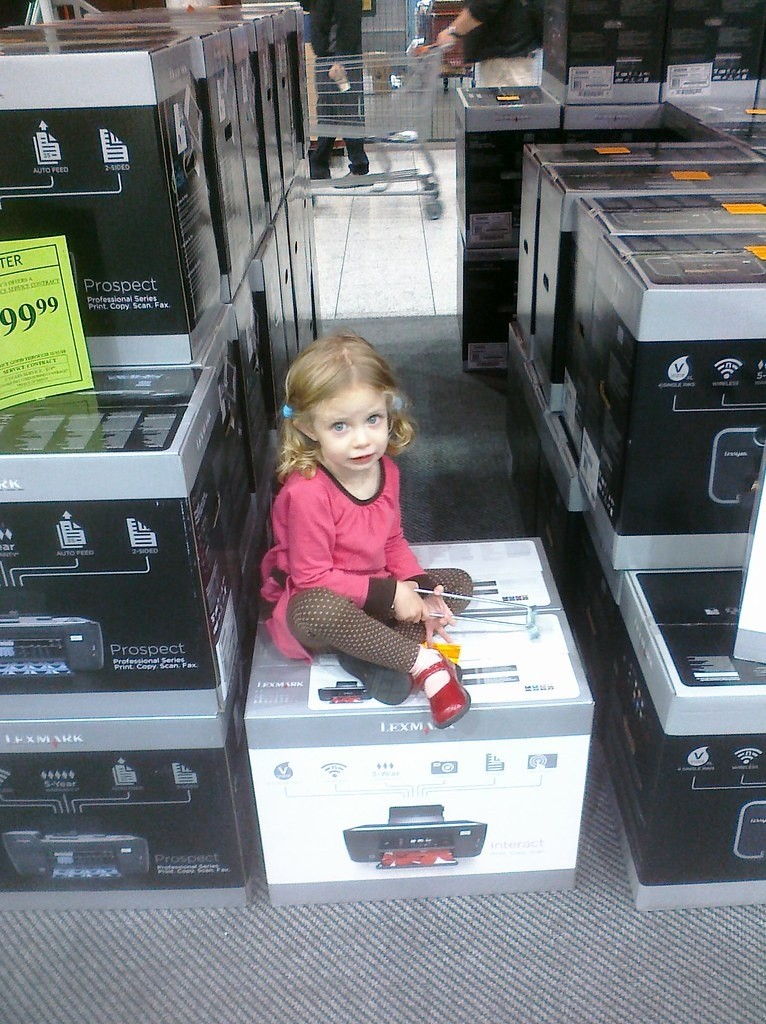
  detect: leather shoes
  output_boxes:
[415,648,471,729]
[336,650,413,706]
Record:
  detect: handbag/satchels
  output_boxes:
[480,5,544,59]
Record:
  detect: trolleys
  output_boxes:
[303,40,458,221]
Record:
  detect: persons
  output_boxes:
[436,0,544,88]
[298,0,391,189]
[260,326,474,730]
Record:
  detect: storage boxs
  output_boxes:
[0,1,766,909]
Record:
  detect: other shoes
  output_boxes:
[335,173,373,189]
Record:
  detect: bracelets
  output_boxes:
[445,25,465,40]
